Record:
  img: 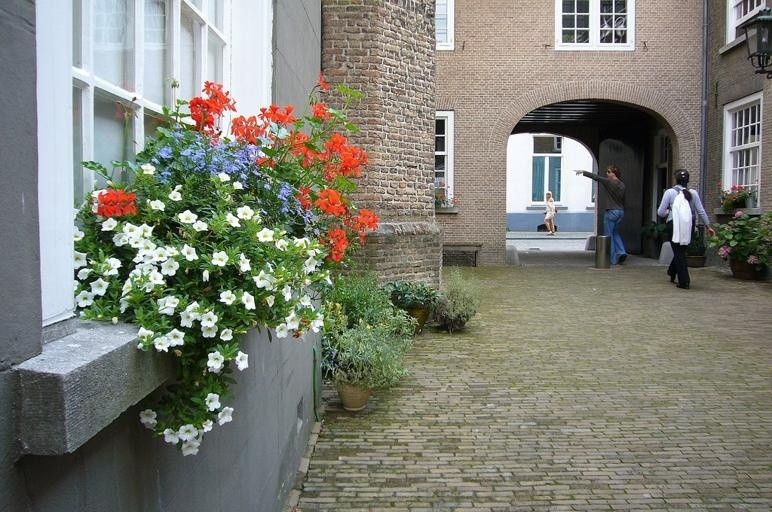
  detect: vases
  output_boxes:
[734,197,747,207]
[729,255,767,281]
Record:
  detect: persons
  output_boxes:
[545,191,558,236]
[572,164,629,267]
[658,169,717,290]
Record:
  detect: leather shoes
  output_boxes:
[668,271,689,289]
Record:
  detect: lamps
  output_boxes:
[736,4,772,80]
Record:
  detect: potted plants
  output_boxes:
[313,264,482,413]
[685,230,707,268]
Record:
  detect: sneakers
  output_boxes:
[616,254,627,263]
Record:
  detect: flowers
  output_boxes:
[717,181,757,208]
[708,210,771,271]
[73,73,377,459]
